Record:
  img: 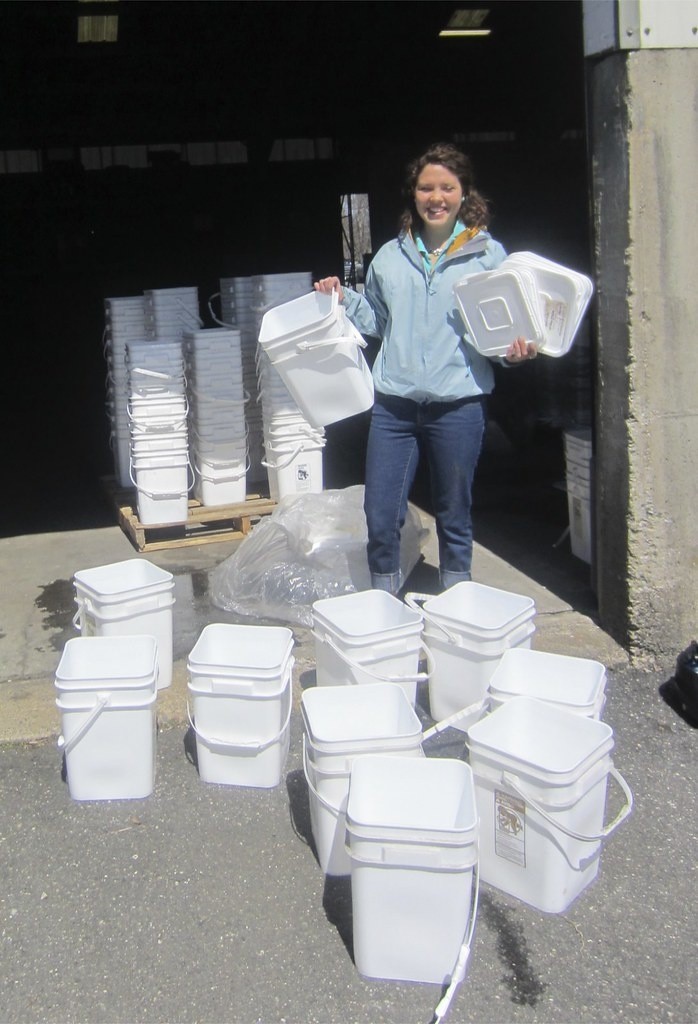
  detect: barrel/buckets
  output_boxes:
[300,683,426,876]
[487,648,607,722]
[344,755,480,1024]
[464,697,633,912]
[187,622,295,787]
[72,558,176,689]
[404,581,535,722]
[563,429,592,564]
[54,635,159,800]
[311,589,436,710]
[104,271,376,524]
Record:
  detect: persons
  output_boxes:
[313,144,537,598]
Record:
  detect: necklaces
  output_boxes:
[425,247,443,256]
